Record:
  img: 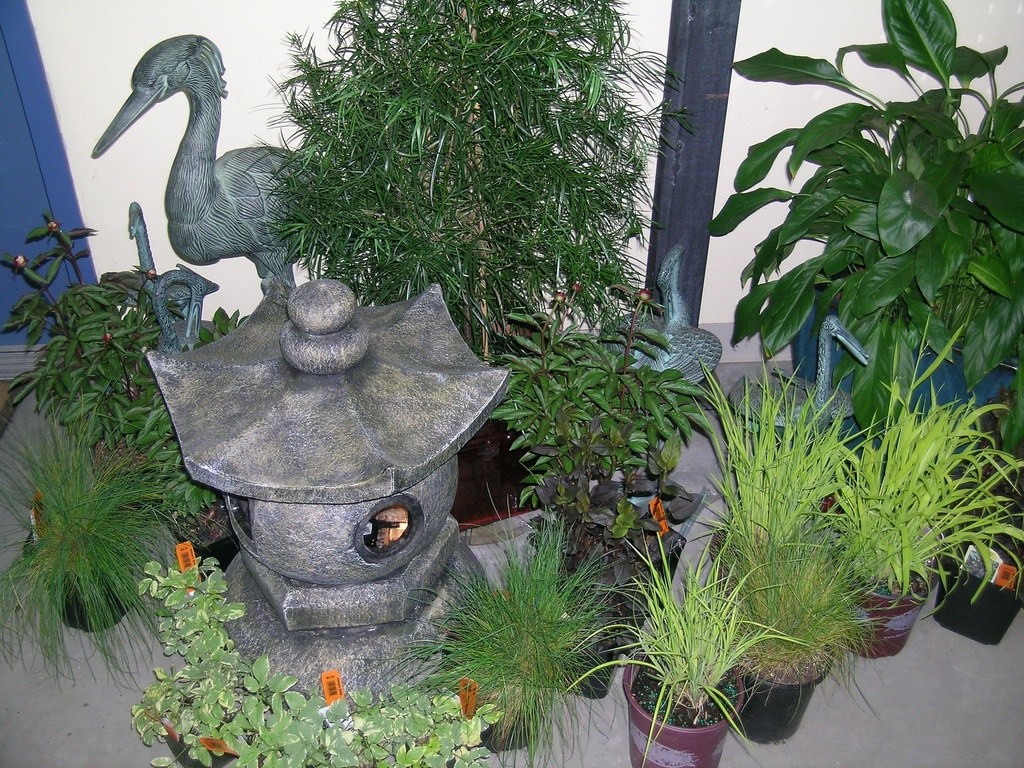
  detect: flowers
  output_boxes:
[597,283,703,521]
[487,281,702,613]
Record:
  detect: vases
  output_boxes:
[933,511,1024,645]
[561,526,687,618]
[627,478,685,513]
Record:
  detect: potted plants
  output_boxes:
[0,212,239,581]
[0,388,239,691]
[558,531,809,768]
[255,0,700,533]
[480,680,543,753]
[819,313,1024,660]
[688,503,900,745]
[236,688,376,768]
[360,482,619,699]
[131,555,265,768]
[687,339,894,592]
[376,686,505,768]
[705,0,1024,487]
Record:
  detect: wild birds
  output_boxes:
[98,199,220,354]
[593,241,723,387]
[92,33,321,292]
[728,313,870,431]
